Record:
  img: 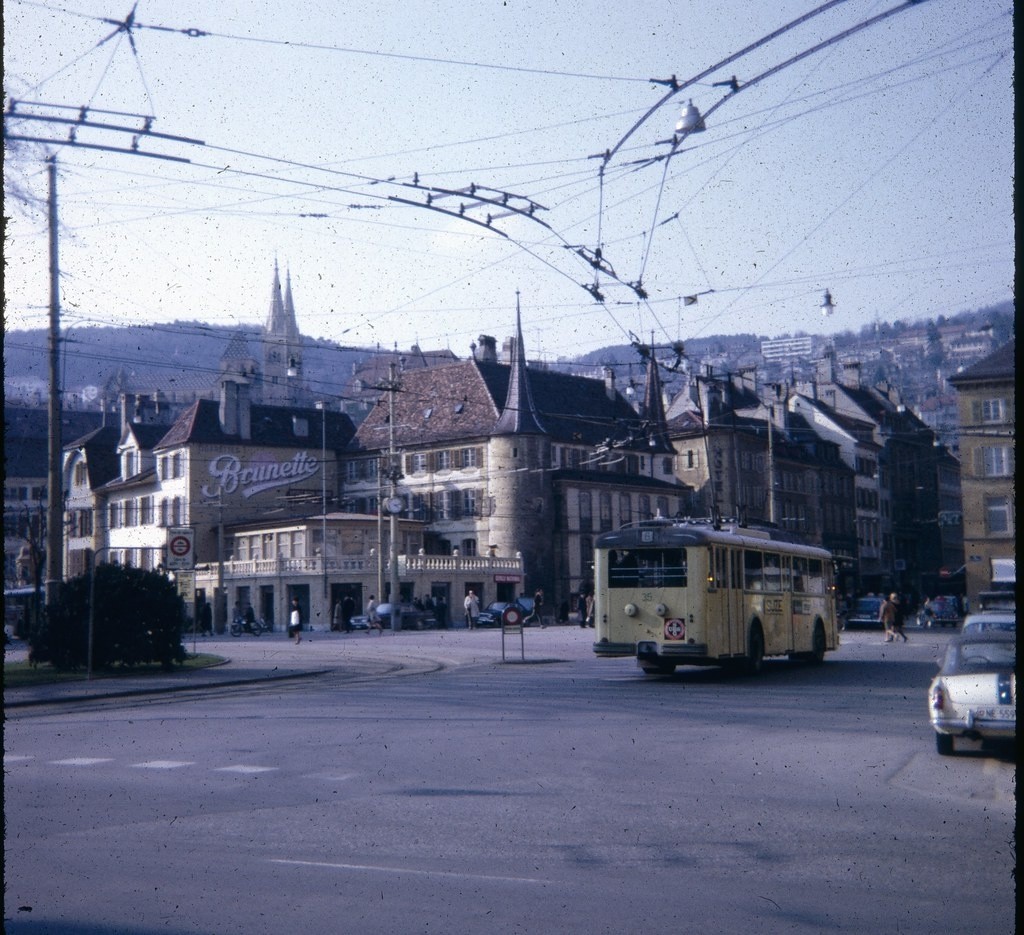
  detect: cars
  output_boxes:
[927,609,1016,754]
[916,601,960,628]
[842,596,886,630]
[477,600,529,627]
[350,603,435,631]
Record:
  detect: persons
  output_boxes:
[413,594,448,629]
[464,590,479,629]
[364,595,384,634]
[331,594,354,633]
[289,597,302,644]
[233,601,255,635]
[924,597,937,629]
[523,587,546,628]
[878,592,909,642]
[837,593,848,630]
[576,592,595,628]
[201,602,214,637]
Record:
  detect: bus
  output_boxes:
[591,506,840,675]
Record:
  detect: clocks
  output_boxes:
[387,497,404,512]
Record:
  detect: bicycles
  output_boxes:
[231,615,263,637]
